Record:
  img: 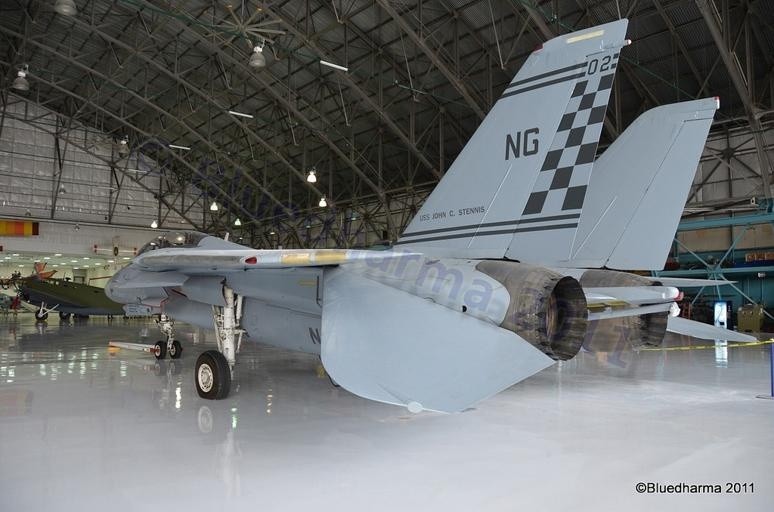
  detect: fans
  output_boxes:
[203,0,286,51]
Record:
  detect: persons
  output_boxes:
[12,296,22,319]
[2,297,9,317]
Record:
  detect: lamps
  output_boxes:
[12,1,30,91]
[248,9,266,69]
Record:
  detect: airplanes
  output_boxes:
[0,262,124,327]
[102,18,758,418]
